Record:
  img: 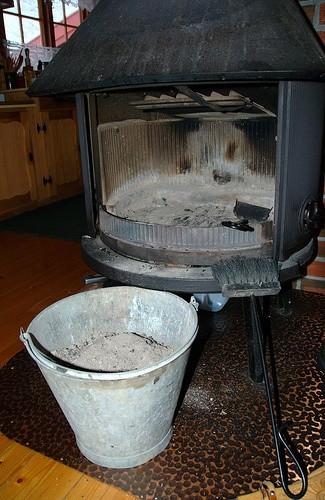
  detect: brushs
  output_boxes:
[212,255,308,500]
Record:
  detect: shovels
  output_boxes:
[222,198,273,232]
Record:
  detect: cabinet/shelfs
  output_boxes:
[0,97,85,220]
